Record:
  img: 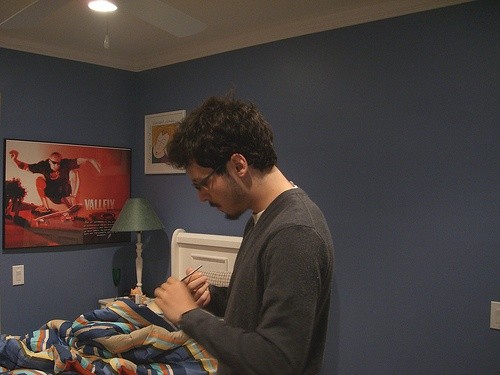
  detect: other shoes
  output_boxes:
[30,207,52,216]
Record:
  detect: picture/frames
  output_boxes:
[3,138,132,250]
[143,110,186,174]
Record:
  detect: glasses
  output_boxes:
[192,155,229,190]
[48,159,61,165]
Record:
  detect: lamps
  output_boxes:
[110,198,166,290]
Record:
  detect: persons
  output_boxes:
[8,148,103,214]
[151,91,337,375]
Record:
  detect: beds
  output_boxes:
[0,228,243,375]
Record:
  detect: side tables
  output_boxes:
[97,295,150,309]
